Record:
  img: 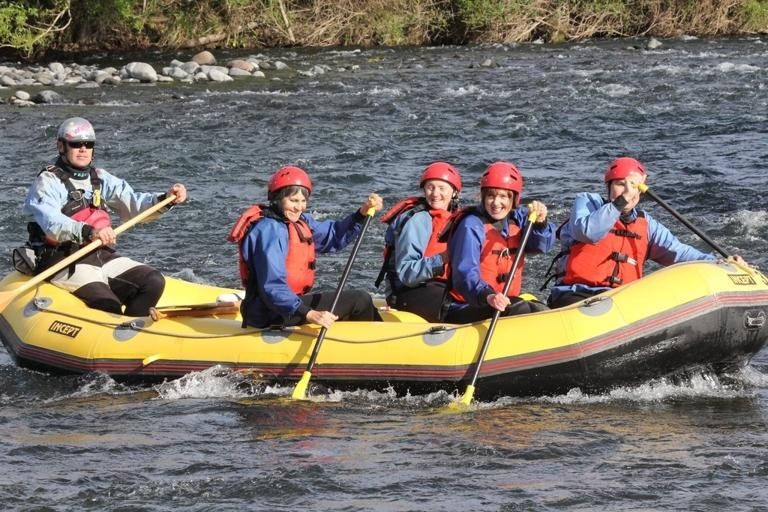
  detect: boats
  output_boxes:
[1,260,768,397]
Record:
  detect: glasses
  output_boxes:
[68,141,95,149]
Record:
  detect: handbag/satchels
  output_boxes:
[397,279,451,323]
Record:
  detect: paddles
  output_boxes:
[152,300,239,308]
[290,210,374,398]
[0,194,177,315]
[147,308,236,318]
[435,214,537,416]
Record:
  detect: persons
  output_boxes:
[23,117,186,318]
[373,162,463,323]
[438,161,557,325]
[228,166,383,329]
[545,156,749,308]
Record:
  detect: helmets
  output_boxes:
[604,157,647,183]
[267,166,313,200]
[57,116,96,145]
[420,162,462,193]
[481,161,522,209]
